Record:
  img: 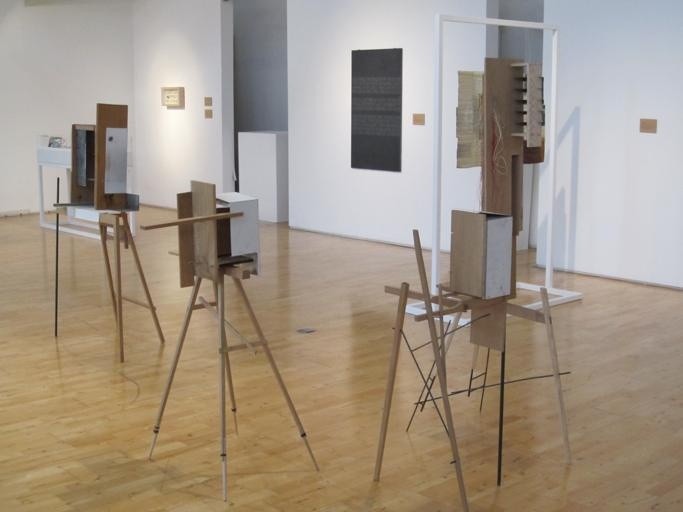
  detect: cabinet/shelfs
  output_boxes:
[34,122,138,244]
[400,10,582,332]
[235,129,289,224]
